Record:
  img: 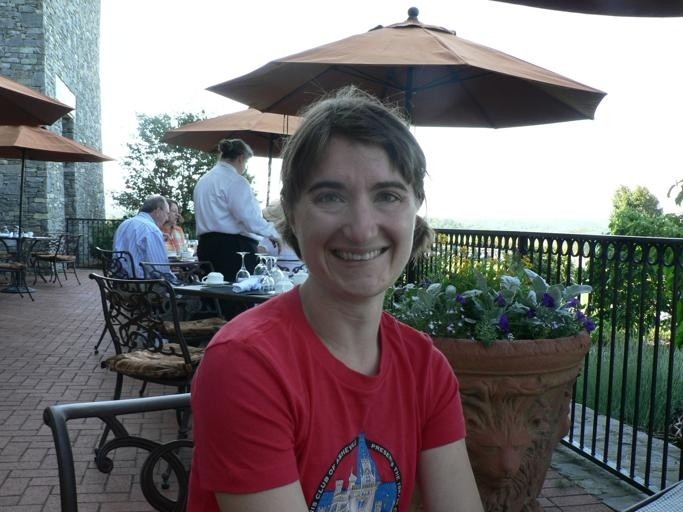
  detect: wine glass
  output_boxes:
[236,252,292,294]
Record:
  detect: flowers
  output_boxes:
[380,233,595,349]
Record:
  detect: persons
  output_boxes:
[114,196,178,300]
[187,96,484,512]
[194,139,283,280]
[258,189,301,260]
[161,199,186,261]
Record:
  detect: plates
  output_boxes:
[196,281,230,287]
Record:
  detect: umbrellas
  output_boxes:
[0,123,112,292]
[205,7,607,131]
[0,76,76,128]
[161,108,335,204]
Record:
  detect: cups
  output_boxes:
[202,272,224,283]
[0,226,34,238]
[293,269,308,284]
[168,250,198,264]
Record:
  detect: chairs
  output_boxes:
[33,234,84,287]
[89,273,208,489]
[139,260,228,396]
[93,246,200,355]
[43,392,191,512]
[0,263,34,301]
[30,233,67,282]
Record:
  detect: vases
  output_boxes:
[432,331,591,512]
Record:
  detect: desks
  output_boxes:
[0,233,53,293]
[171,281,294,303]
[167,252,299,267]
